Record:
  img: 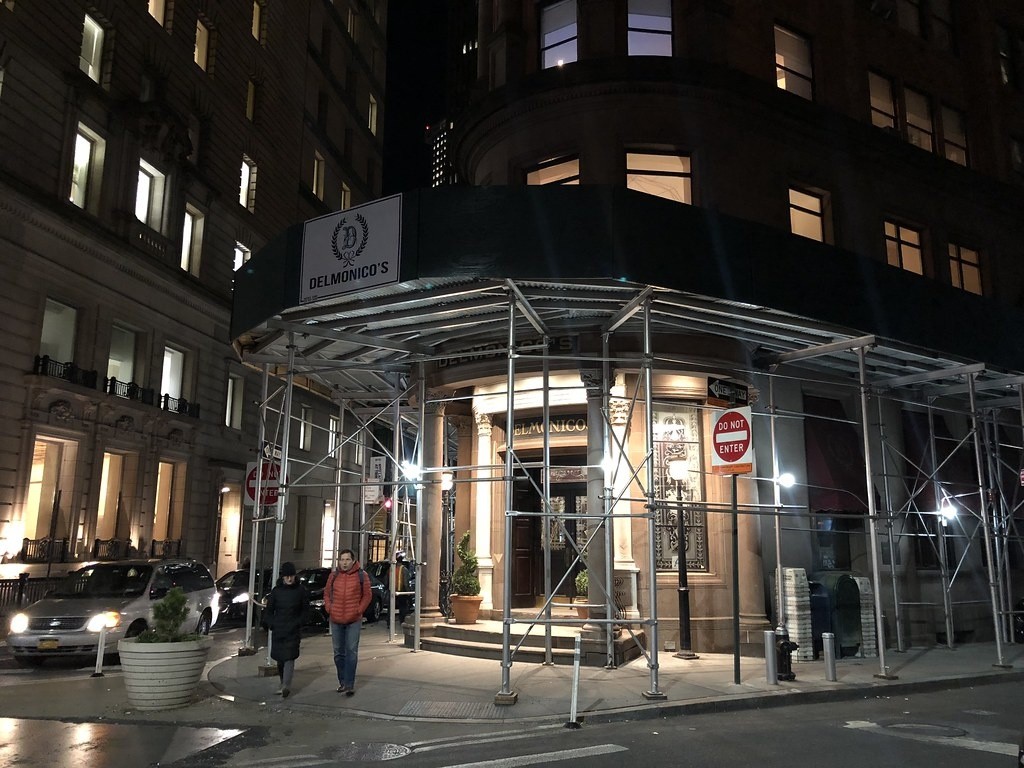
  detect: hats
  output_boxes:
[278,562,296,578]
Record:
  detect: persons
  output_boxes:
[323,549,371,696]
[388,550,411,630]
[261,561,308,702]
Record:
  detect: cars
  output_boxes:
[363,560,414,593]
[5,558,218,662]
[259,568,385,631]
[213,569,270,617]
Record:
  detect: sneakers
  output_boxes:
[345,688,355,697]
[274,684,282,694]
[282,687,290,698]
[337,685,346,693]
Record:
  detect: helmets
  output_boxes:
[395,550,407,560]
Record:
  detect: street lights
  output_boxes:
[441,465,455,591]
[667,453,699,661]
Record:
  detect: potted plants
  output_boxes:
[572,568,589,619]
[117,586,215,713]
[449,529,483,624]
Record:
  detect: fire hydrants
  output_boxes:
[775,626,799,681]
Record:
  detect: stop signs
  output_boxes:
[708,407,753,474]
[243,461,290,506]
